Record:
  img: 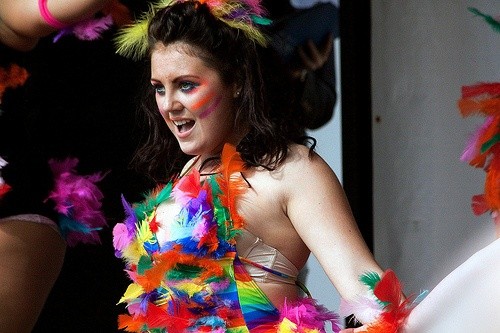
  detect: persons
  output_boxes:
[0,1,113,333]
[112,1,413,333]
